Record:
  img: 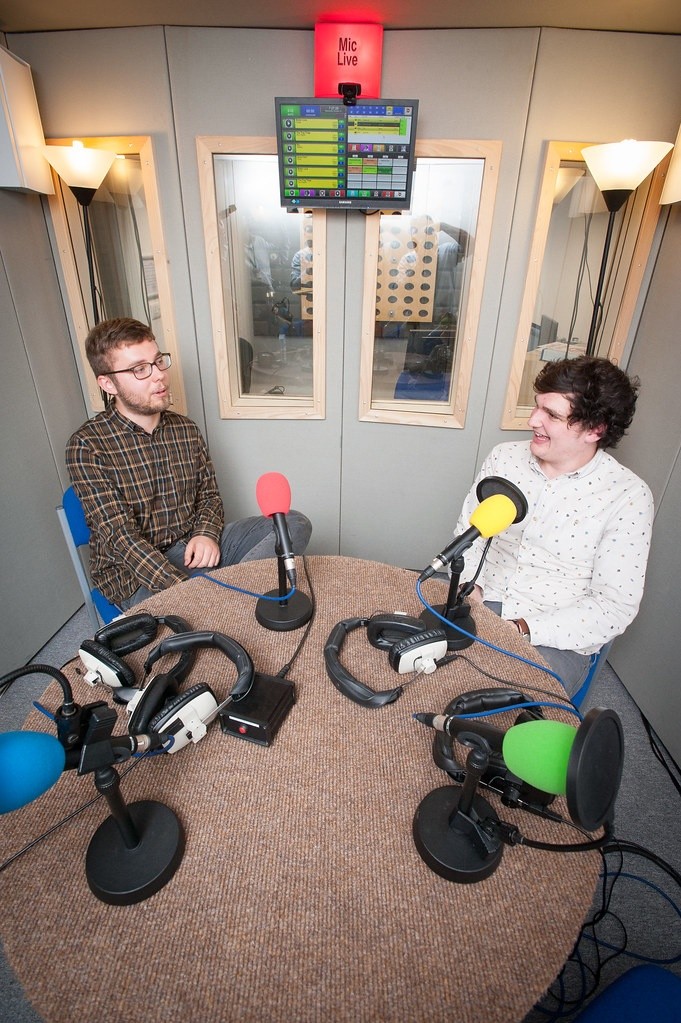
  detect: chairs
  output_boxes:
[570,641,612,709]
[57,484,120,633]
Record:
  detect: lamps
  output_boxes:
[581,138,674,356]
[41,140,120,410]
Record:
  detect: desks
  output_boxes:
[0,555,602,1023]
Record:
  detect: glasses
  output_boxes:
[102,354,172,380]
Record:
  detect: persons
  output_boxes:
[65,316,313,614]
[289,223,313,295]
[400,216,473,319]
[447,357,654,699]
[243,207,290,289]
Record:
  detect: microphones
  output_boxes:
[0,731,170,814]
[257,472,297,588]
[413,711,578,795]
[418,492,518,583]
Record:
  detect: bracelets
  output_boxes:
[509,617,530,642]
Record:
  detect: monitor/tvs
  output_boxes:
[275,97,419,211]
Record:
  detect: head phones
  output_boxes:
[432,687,556,809]
[126,629,255,753]
[78,613,199,703]
[324,610,447,709]
